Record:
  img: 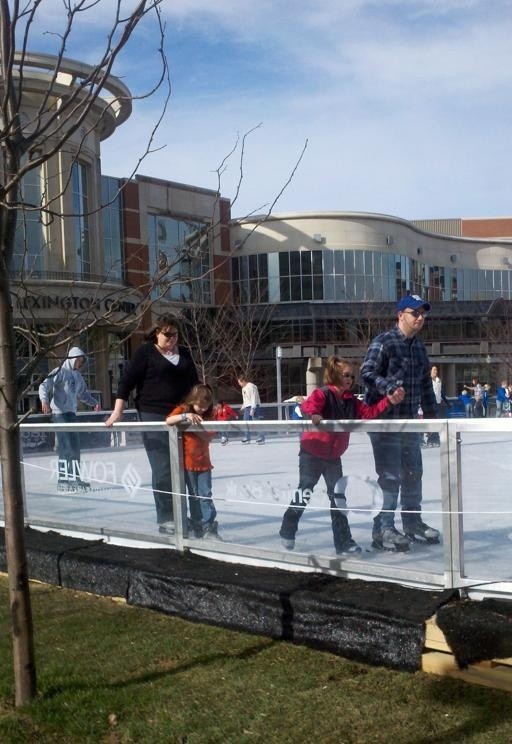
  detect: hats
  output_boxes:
[397,295,432,311]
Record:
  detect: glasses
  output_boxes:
[160,331,180,338]
[340,371,355,379]
[405,311,429,318]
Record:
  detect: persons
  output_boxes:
[38,347,101,494]
[213,400,238,445]
[104,313,203,537]
[166,384,221,542]
[460,378,510,418]
[237,376,266,444]
[292,397,305,420]
[359,295,440,552]
[278,355,405,557]
[426,367,443,446]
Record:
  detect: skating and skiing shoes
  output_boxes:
[421,435,440,447]
[281,535,295,550]
[404,521,440,544]
[373,524,412,553]
[335,539,362,557]
[160,516,221,541]
[241,436,251,444]
[58,478,90,492]
[256,438,265,445]
[220,435,228,446]
